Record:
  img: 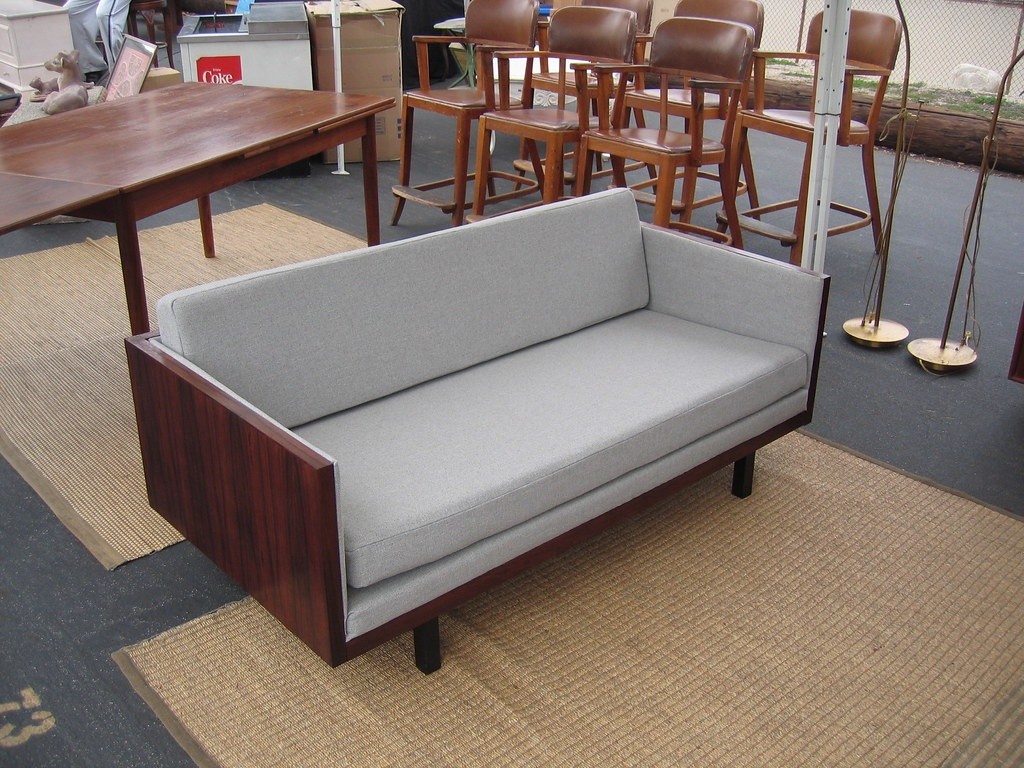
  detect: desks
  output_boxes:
[0,81,397,333]
[434,16,477,89]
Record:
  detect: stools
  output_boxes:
[129,0,176,71]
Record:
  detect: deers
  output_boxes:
[41,48,89,115]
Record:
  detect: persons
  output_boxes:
[62,0,133,83]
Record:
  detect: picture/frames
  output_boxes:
[96,34,159,105]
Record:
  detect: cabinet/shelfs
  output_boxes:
[0,0,74,86]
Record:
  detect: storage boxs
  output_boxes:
[144,66,181,94]
[306,0,404,165]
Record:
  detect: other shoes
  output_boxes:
[85,67,110,87]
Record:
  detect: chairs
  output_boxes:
[391,1,903,268]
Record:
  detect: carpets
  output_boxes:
[1,201,382,573]
[111,425,1024,767]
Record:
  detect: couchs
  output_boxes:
[124,185,829,675]
